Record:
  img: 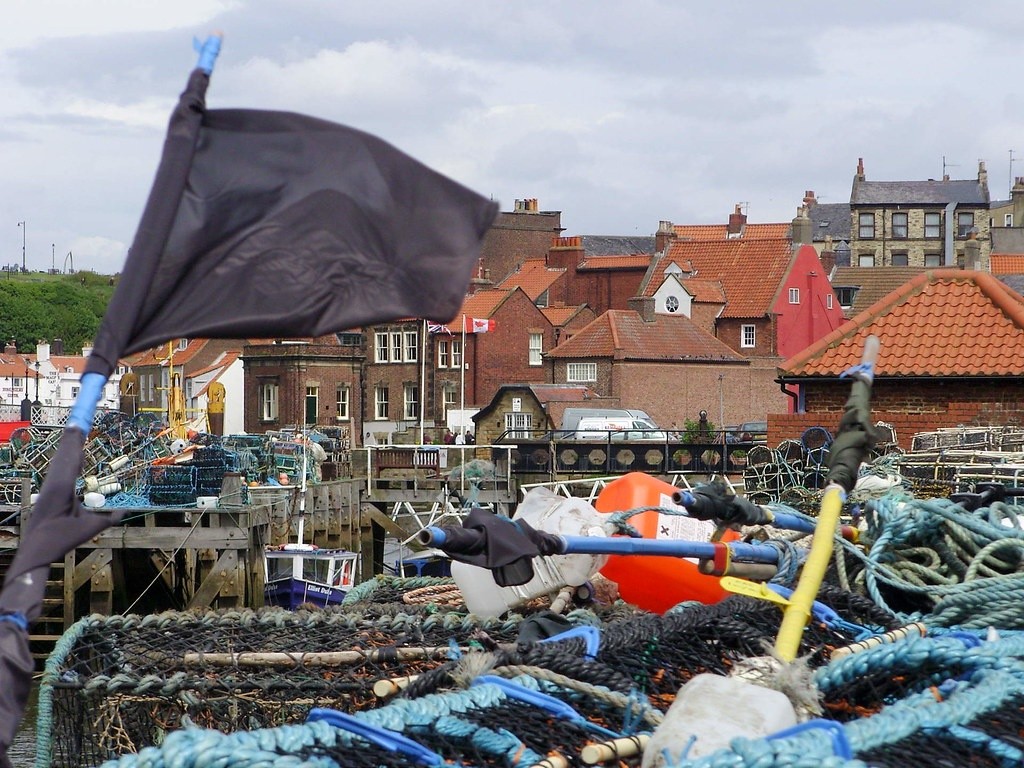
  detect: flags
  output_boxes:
[463,317,497,334]
[78,69,502,378]
[426,320,453,336]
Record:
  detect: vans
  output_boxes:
[575,416,669,440]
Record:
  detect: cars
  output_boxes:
[715,422,766,444]
